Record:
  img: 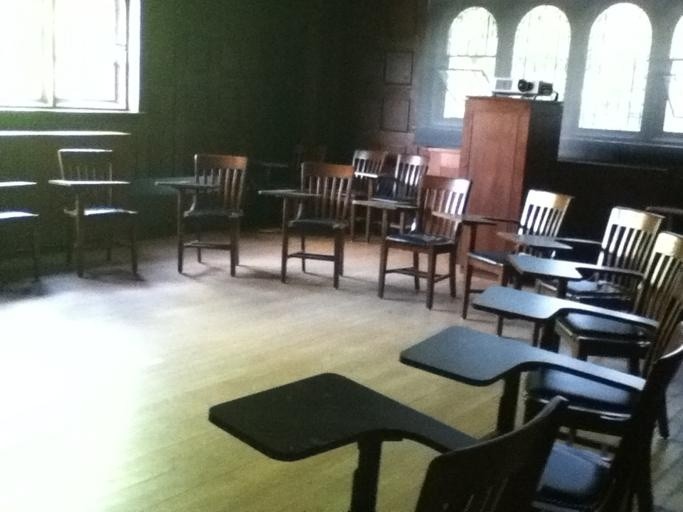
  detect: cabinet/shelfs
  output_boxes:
[459,95,564,283]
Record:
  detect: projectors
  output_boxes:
[492,76,554,97]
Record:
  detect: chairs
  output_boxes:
[352,174,473,311]
[256,161,353,290]
[47,147,140,279]
[399,324,683,511]
[346,150,388,200]
[155,153,248,277]
[473,230,683,440]
[431,188,576,336]
[506,205,665,353]
[0,178,41,284]
[350,152,430,245]
[208,371,568,511]
